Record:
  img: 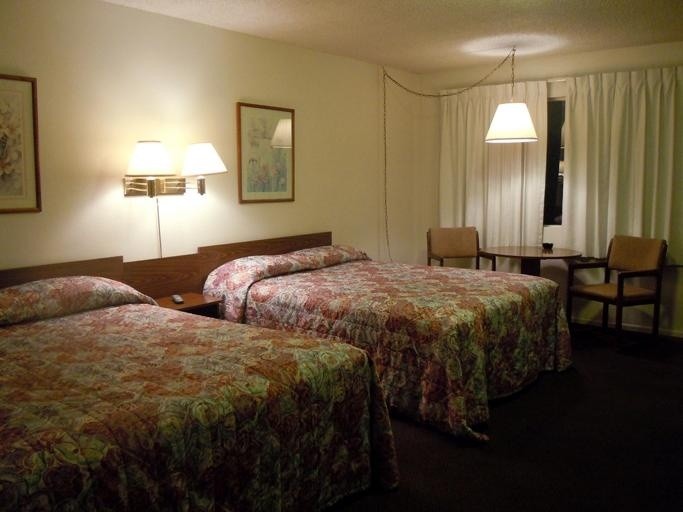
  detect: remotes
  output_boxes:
[172,294,184,303]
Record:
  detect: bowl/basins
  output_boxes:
[542,249,553,254]
[542,242,553,249]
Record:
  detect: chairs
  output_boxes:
[572,233,667,348]
[423,227,494,273]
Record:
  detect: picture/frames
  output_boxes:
[0,72,43,218]
[234,99,296,206]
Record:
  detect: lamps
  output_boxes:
[122,139,229,199]
[481,45,541,147]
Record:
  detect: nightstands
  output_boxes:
[150,292,224,317]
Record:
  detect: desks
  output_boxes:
[478,245,581,280]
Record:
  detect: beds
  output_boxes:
[197,227,572,445]
[1,256,404,512]
